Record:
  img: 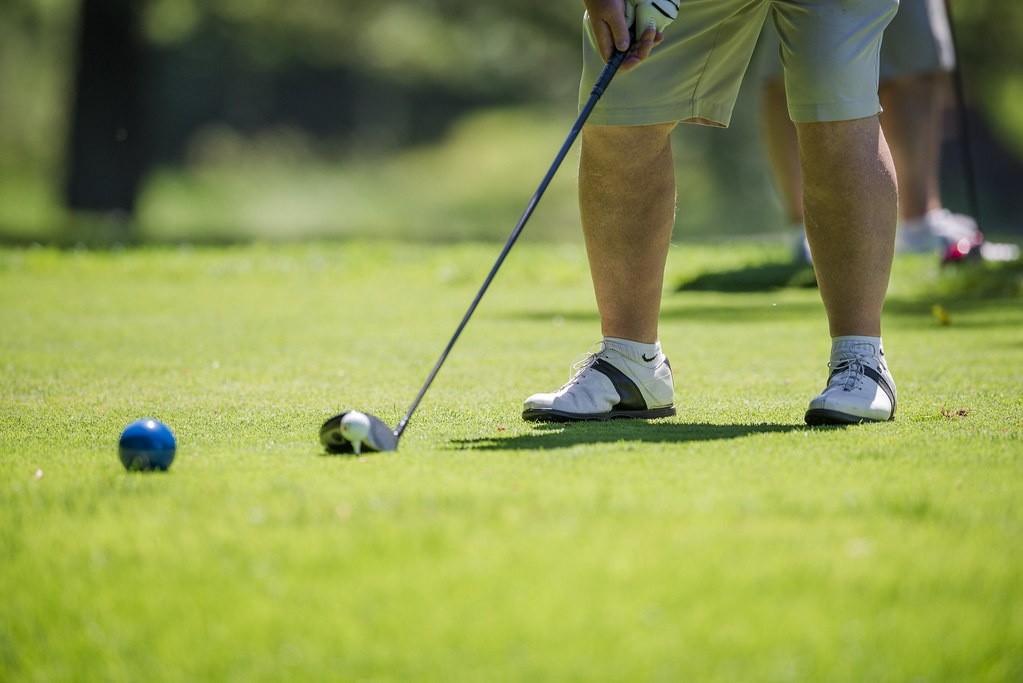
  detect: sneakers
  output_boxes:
[521,340,676,421]
[804,347,898,425]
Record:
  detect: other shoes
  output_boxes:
[896,208,976,255]
[787,244,816,287]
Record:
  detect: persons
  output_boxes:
[760,1,982,284]
[520,0,905,422]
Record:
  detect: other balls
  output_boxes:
[119,419,176,473]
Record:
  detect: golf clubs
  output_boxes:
[320,23,637,453]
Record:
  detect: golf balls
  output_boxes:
[340,411,370,442]
